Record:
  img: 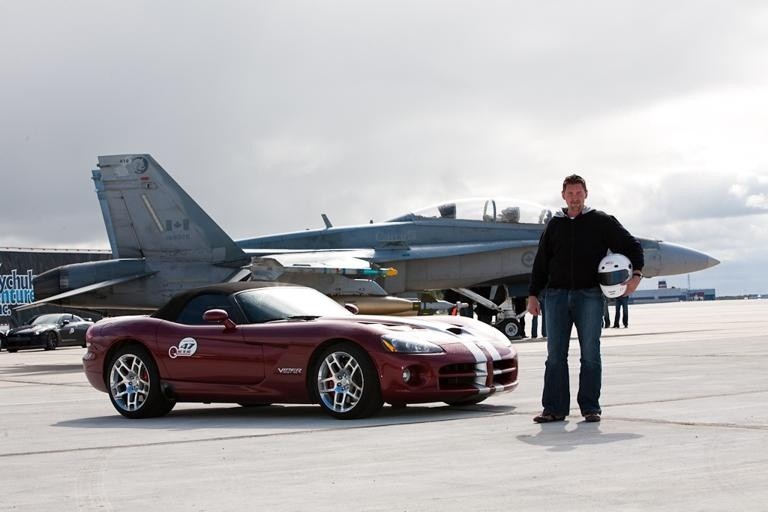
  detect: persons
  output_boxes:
[527,174,645,423]
[442,288,629,340]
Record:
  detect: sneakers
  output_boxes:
[584,412,601,423]
[532,410,567,423]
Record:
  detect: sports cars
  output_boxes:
[80,277,522,420]
[3,310,97,353]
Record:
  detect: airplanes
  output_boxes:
[20,149,723,338]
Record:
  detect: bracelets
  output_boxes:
[632,272,644,279]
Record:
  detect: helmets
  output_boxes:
[597,254,634,300]
